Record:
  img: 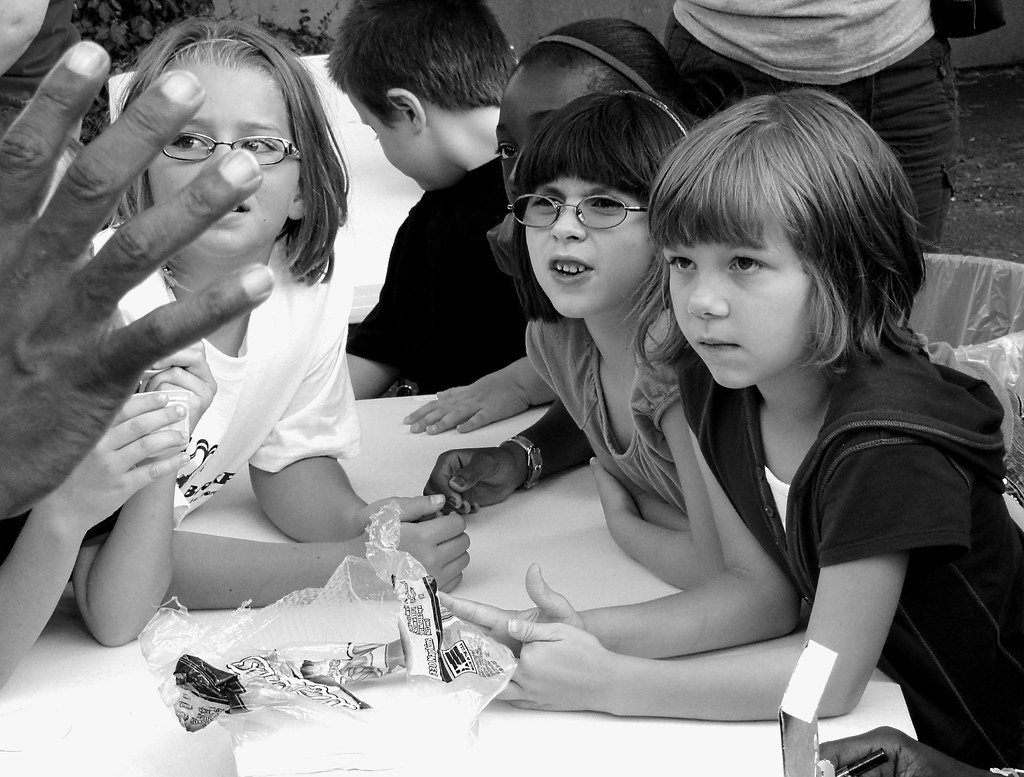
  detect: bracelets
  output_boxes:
[500,436,544,491]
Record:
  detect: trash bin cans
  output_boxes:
[874,251,1023,421]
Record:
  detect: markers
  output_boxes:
[832,748,889,777]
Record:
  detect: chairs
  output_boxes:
[893,248,1024,428]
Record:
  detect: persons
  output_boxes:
[0,137,219,651]
[821,726,1024,776]
[0,38,279,522]
[430,87,1024,777]
[325,2,560,438]
[506,87,729,593]
[2,0,87,151]
[423,15,713,519]
[665,0,959,253]
[87,10,473,613]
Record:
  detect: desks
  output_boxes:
[1,52,920,776]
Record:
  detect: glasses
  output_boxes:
[160,131,302,165]
[507,192,652,230]
